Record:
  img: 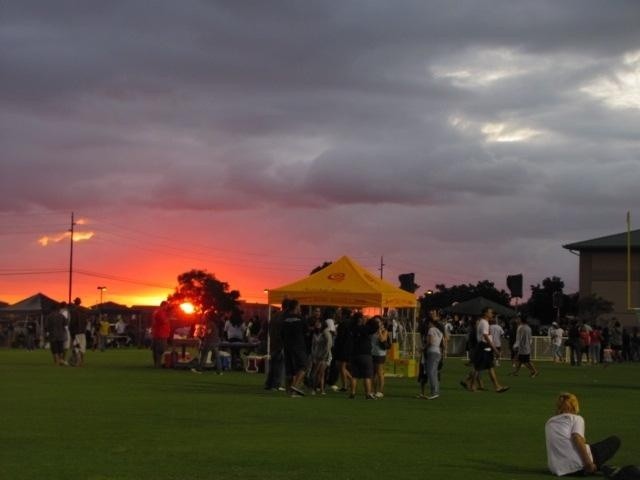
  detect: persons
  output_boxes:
[7,314,41,352]
[264,301,392,400]
[415,305,538,400]
[548,318,639,365]
[389,315,418,353]
[153,302,265,373]
[46,297,87,368]
[87,313,153,352]
[545,391,638,478]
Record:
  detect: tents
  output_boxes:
[4,292,60,348]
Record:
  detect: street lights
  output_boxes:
[97,287,107,321]
[428,289,433,301]
[265,289,271,294]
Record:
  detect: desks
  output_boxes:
[167,339,260,370]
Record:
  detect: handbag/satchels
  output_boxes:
[469,341,494,364]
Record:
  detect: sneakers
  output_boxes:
[339,388,384,399]
[600,463,619,476]
[264,383,327,398]
[417,392,439,398]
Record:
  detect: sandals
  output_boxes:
[460,381,512,393]
